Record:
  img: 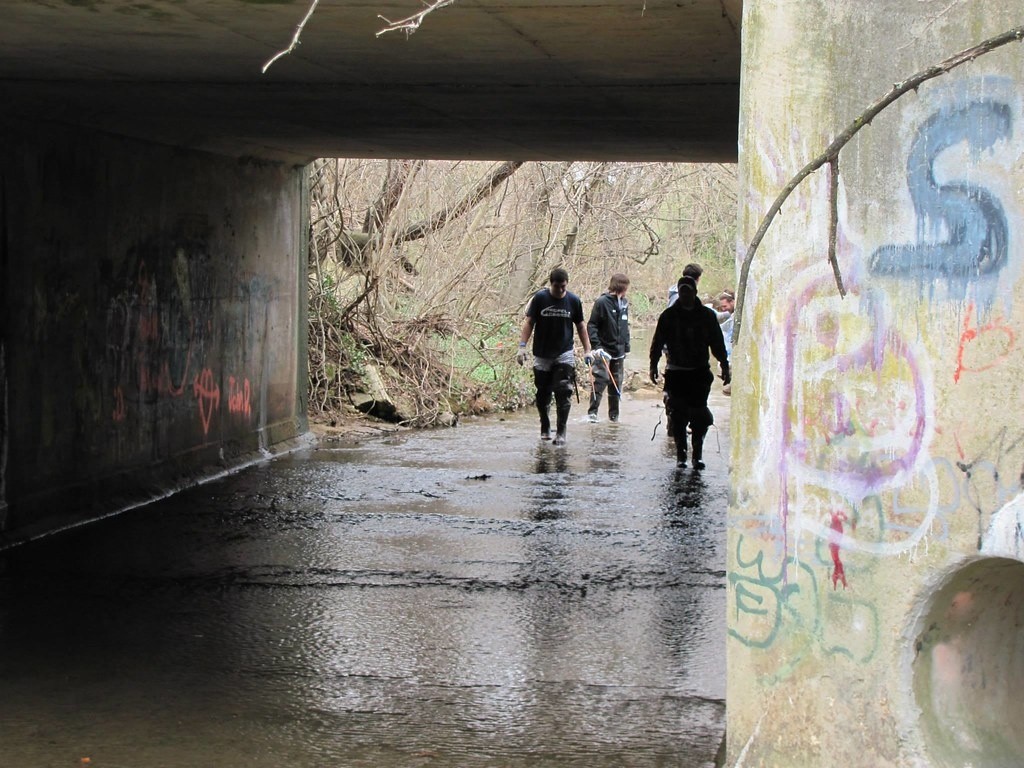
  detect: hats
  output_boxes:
[678,276,697,292]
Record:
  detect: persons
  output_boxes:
[517,269,592,445]
[588,274,631,425]
[650,276,730,469]
[663,263,735,445]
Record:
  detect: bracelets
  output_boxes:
[520,342,526,347]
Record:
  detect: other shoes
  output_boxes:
[537,405,552,440]
[552,406,572,444]
[691,433,705,470]
[608,394,620,421]
[588,390,602,423]
[674,437,687,469]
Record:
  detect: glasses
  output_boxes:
[722,291,732,297]
